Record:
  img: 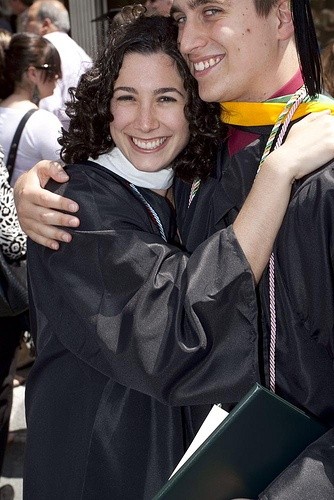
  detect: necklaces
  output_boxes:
[164,185,174,200]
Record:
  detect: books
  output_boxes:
[152,382,330,500]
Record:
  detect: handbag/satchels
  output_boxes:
[0,252,29,316]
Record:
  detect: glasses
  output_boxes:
[36,64,57,73]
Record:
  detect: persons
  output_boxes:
[0,1,94,479]
[23,27,334,500]
[14,1,334,498]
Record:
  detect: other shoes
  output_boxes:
[0,484,14,500]
